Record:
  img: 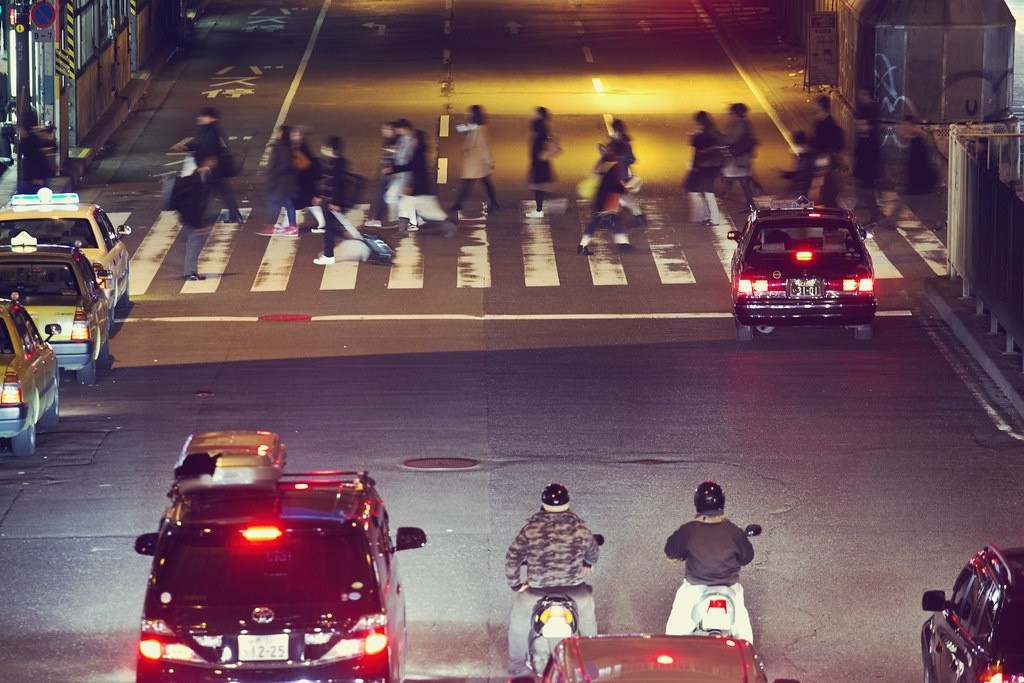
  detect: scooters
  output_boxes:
[511,532,603,651]
[688,523,771,645]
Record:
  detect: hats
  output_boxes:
[198,107,221,119]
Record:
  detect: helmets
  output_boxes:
[694,482,725,514]
[542,483,571,513]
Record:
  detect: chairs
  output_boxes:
[38,280,68,295]
[59,223,91,245]
[822,230,846,250]
[765,230,794,251]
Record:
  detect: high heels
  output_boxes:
[578,245,594,255]
[631,214,649,229]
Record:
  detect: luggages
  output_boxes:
[331,207,398,265]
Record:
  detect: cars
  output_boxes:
[1,188,131,333]
[0,293,64,453]
[1,242,116,386]
[172,428,288,482]
[509,634,798,683]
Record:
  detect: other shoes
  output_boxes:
[740,201,756,213]
[408,222,419,231]
[364,218,382,227]
[257,226,276,236]
[282,227,301,237]
[526,210,544,218]
[314,254,335,265]
[222,217,243,225]
[183,273,210,281]
[703,220,721,227]
[312,225,327,234]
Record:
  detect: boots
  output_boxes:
[440,219,458,241]
[394,218,410,239]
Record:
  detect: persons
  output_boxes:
[163,81,941,283]
[507,483,600,672]
[965,136,1001,179]
[14,100,59,200]
[660,478,754,644]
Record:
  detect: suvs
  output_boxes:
[130,468,427,683]
[917,542,1024,683]
[727,199,879,341]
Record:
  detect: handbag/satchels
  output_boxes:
[164,177,180,210]
[702,144,731,168]
[622,174,643,192]
[540,138,563,161]
